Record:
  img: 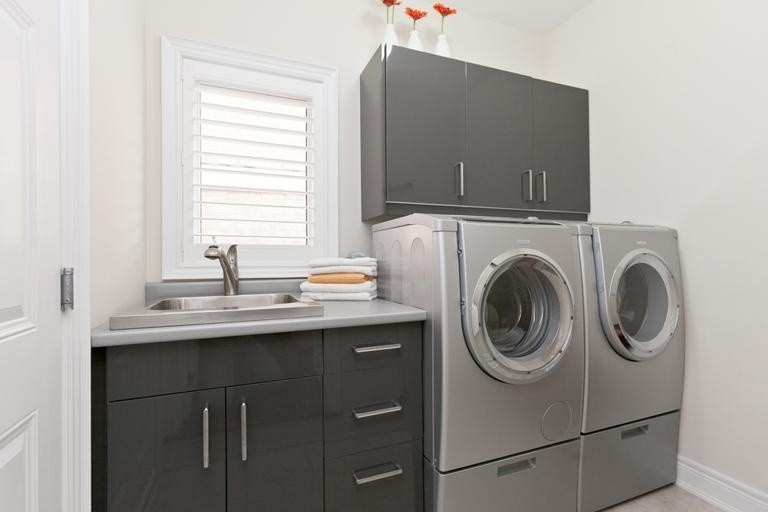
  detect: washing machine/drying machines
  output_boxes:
[371,213,585,511]
[577,221,687,512]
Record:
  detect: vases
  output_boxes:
[381,26,454,60]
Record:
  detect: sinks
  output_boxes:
[108,292,324,332]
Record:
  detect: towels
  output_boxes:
[297,256,378,299]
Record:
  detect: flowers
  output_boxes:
[382,0,457,34]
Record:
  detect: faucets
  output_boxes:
[203,243,240,294]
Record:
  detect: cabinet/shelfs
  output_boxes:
[467,62,590,223]
[322,314,425,512]
[361,41,467,222]
[89,332,322,512]
